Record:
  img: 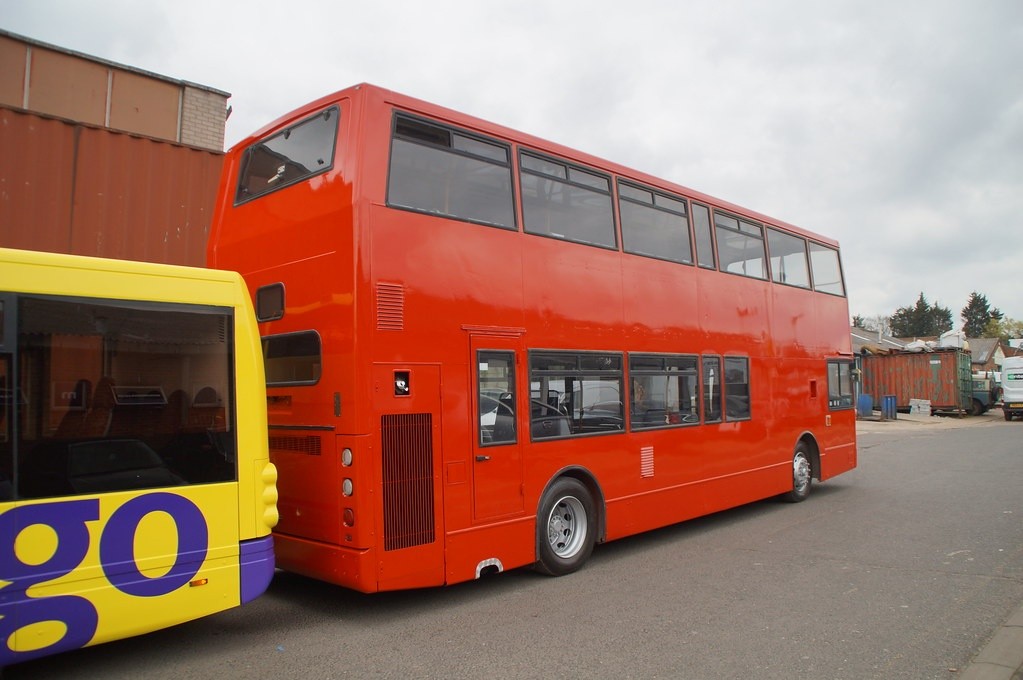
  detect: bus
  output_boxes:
[205,82,860,593]
[0,247,280,667]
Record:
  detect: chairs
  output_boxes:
[0,380,228,441]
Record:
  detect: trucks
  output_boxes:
[1001,355,1023,420]
[966,370,1002,416]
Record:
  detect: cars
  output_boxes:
[477,382,750,443]
